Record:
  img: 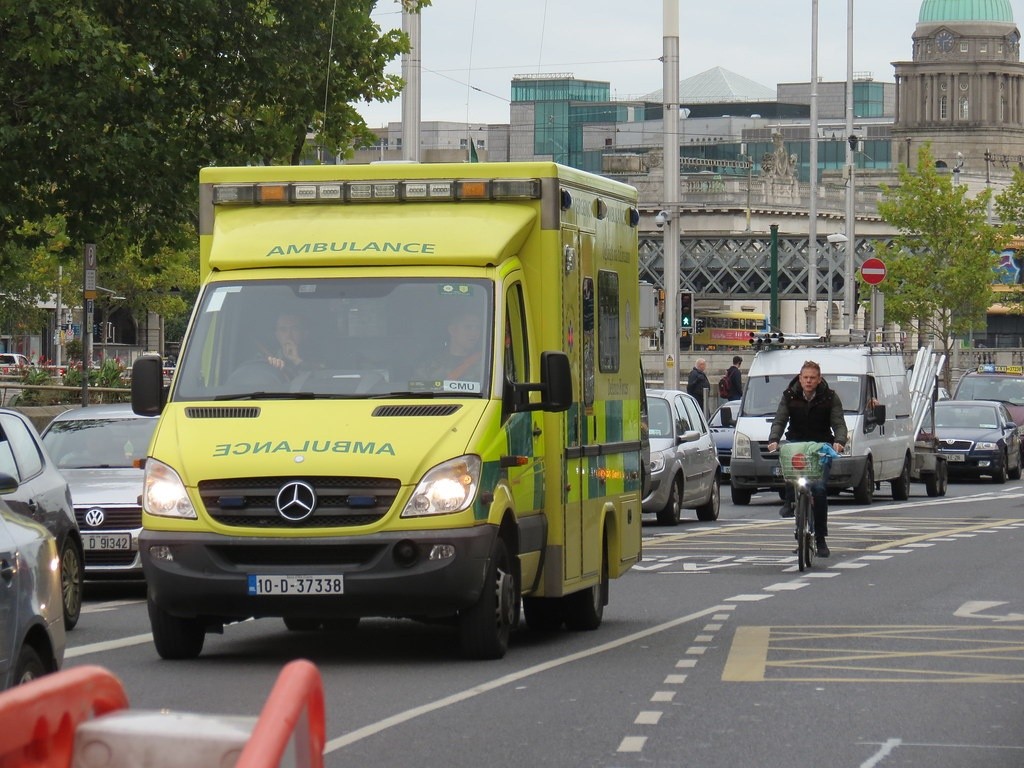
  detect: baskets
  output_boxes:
[776,453,826,481]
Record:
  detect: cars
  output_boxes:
[918,401,1021,484]
[937,387,951,401]
[642,388,720,525]
[0,354,34,375]
[38,404,161,597]
[709,399,742,484]
[0,411,84,690]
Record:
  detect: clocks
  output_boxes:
[935,31,954,51]
[1008,33,1019,50]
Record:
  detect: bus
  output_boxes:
[694,311,768,345]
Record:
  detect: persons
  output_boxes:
[727,356,742,401]
[768,361,847,557]
[232,304,321,393]
[687,358,711,420]
[423,300,484,384]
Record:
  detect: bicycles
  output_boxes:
[771,445,845,572]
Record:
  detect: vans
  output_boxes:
[730,345,915,505]
[951,369,1024,468]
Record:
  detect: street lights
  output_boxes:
[827,233,855,328]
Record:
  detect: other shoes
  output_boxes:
[778,497,794,518]
[816,536,830,559]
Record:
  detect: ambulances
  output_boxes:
[129,158,639,661]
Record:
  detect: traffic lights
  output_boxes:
[680,292,693,329]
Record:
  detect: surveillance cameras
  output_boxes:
[655,214,665,227]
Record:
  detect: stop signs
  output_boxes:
[861,258,886,286]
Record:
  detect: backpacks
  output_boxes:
[718,367,737,399]
